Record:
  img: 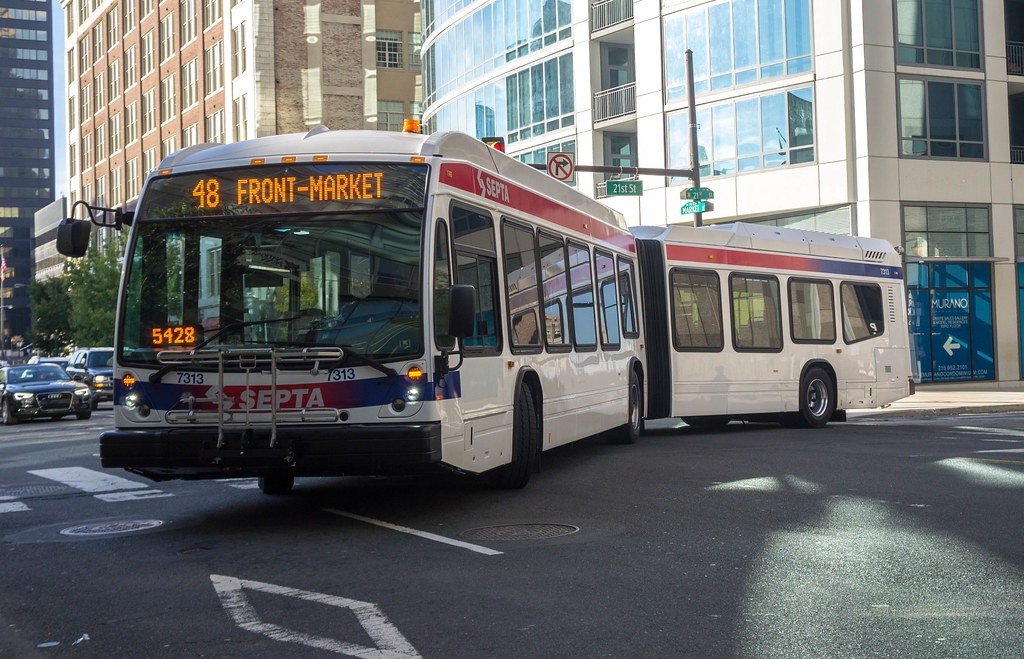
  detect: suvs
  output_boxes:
[65,345,148,411]
[20,355,69,380]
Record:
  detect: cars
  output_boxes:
[0,363,94,425]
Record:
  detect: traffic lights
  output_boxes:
[480,136,505,154]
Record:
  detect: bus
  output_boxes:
[53,119,916,491]
